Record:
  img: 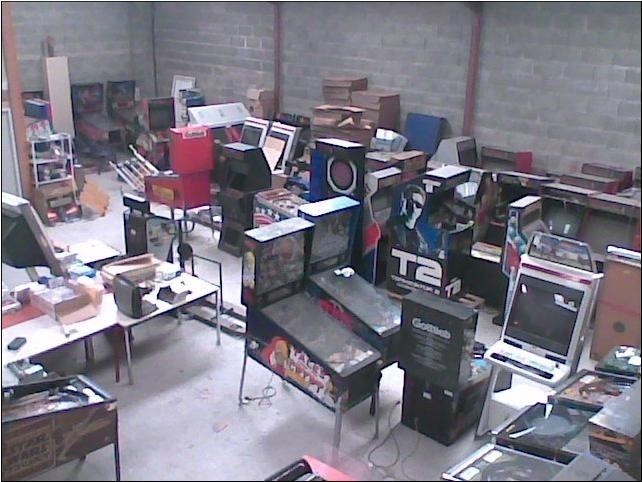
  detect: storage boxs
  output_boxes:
[310,76,400,151]
[248,102,274,118]
[247,84,274,101]
[30,287,98,324]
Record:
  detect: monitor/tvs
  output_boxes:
[542,199,581,237]
[581,210,636,257]
[2,190,65,277]
[242,125,263,146]
[504,273,584,358]
[459,150,477,163]
[482,158,511,173]
[150,105,171,128]
[269,131,290,167]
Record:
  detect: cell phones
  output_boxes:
[8,336,27,350]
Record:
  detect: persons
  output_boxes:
[387,183,427,256]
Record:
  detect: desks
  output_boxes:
[87,262,220,385]
[2,275,121,382]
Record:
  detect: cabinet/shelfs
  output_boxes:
[27,133,73,190]
